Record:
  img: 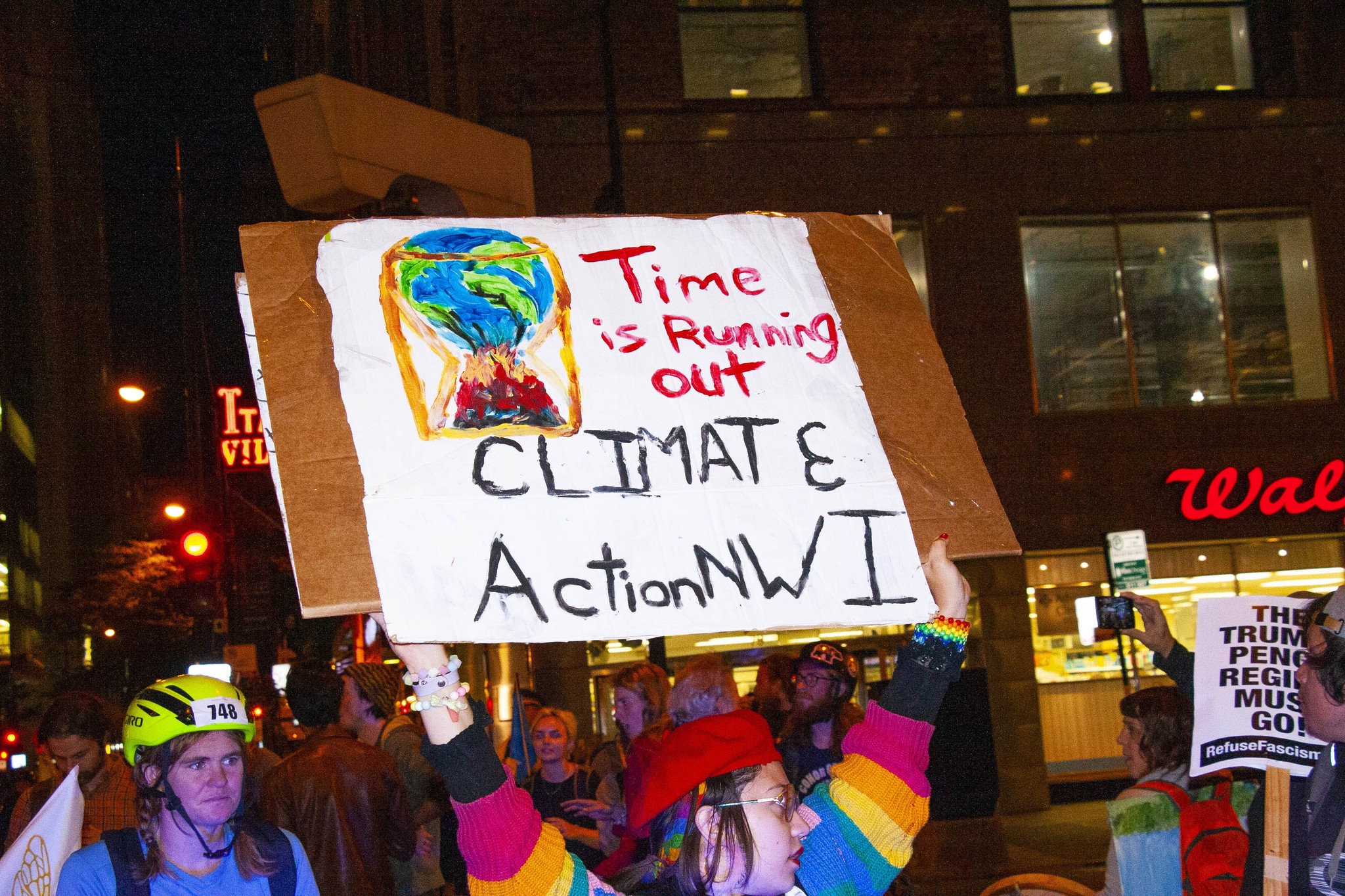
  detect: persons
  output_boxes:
[1093,685,1233,896]
[258,657,415,896]
[561,658,672,896]
[364,532,972,896]
[1120,584,1345,896]
[55,674,322,896]
[515,707,608,873]
[338,663,435,896]
[520,688,546,726]
[774,640,866,809]
[5,693,138,849]
[753,653,799,748]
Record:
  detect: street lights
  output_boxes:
[117,379,241,670]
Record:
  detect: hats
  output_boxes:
[519,689,544,709]
[1314,585,1345,639]
[345,662,401,714]
[592,707,782,885]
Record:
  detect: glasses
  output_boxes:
[712,783,800,822]
[790,670,836,687]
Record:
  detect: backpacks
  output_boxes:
[1126,769,1249,896]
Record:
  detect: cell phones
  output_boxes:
[1095,595,1136,630]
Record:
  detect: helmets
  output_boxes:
[122,673,255,767]
[795,640,859,698]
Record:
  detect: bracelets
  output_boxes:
[400,654,471,722]
[911,615,971,653]
